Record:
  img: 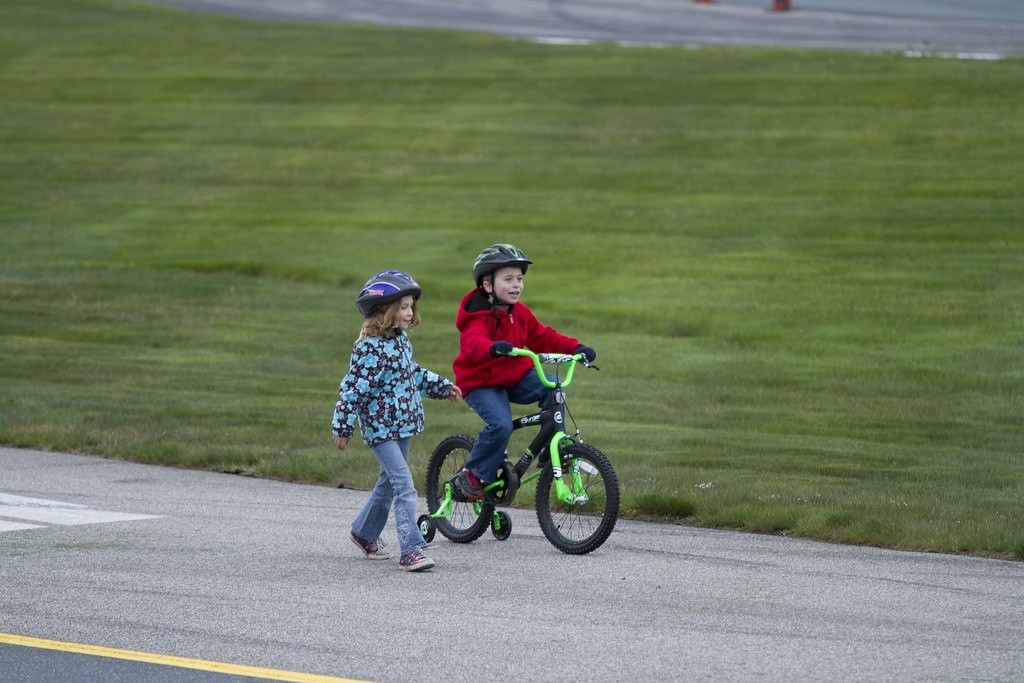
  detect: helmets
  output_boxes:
[355,270,422,317]
[472,244,533,306]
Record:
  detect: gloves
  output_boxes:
[573,345,596,362]
[490,340,513,359]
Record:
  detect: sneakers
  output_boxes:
[399,546,434,572]
[349,529,393,559]
[454,467,483,499]
[536,454,570,468]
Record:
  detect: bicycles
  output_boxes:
[417,348,620,555]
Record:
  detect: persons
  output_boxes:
[452,244,596,500]
[331,271,462,573]
